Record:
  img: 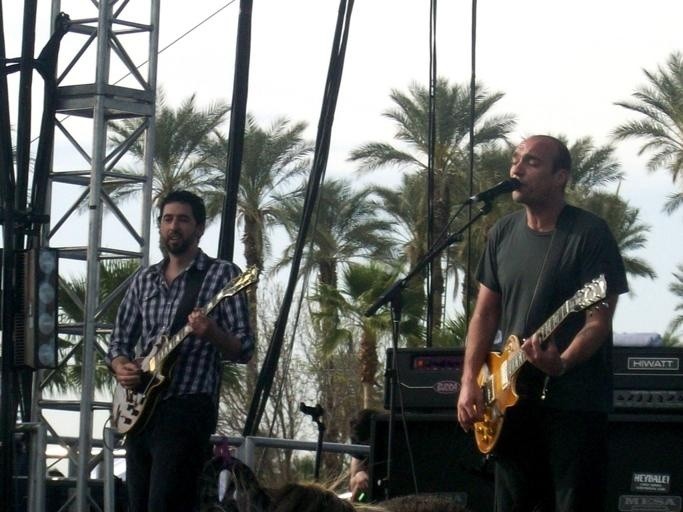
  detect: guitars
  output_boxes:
[473,275,609,454]
[112,265,261,437]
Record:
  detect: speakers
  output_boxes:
[369,347,683,511]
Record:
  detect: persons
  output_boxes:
[106,187,256,512]
[454,133,629,512]
[347,408,383,503]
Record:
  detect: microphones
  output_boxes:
[469,177,520,205]
